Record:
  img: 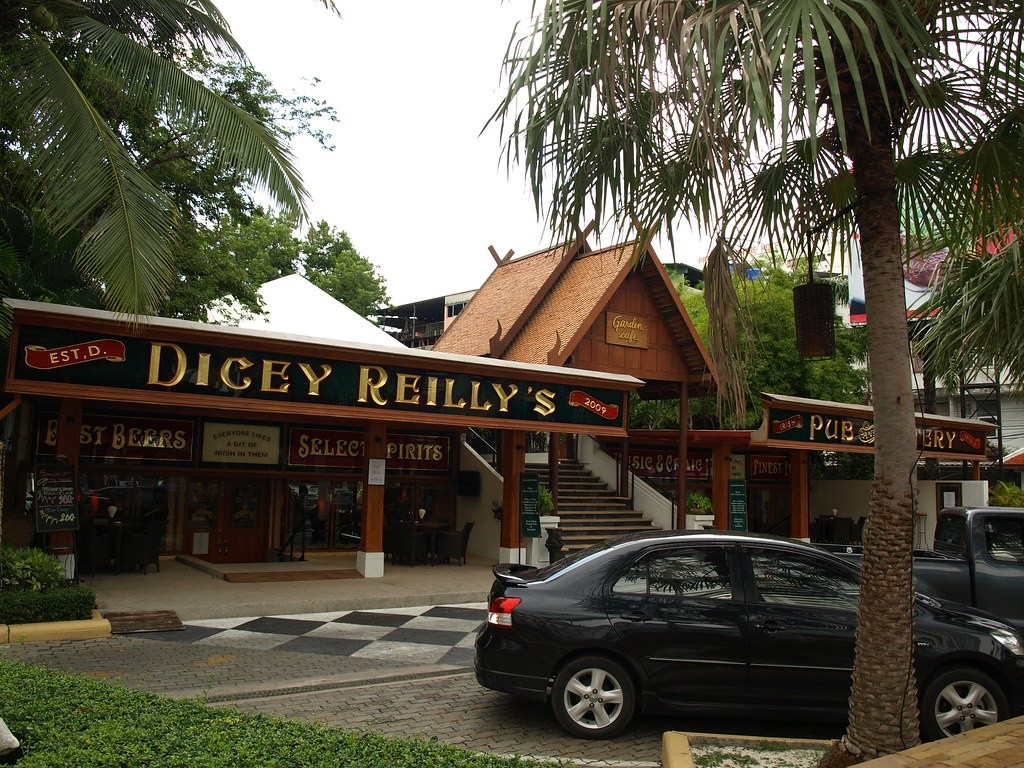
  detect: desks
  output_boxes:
[92,519,144,575]
[814,516,831,543]
[409,522,449,564]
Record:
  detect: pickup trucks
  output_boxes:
[805,506,1024,637]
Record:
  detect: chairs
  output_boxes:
[383,528,396,563]
[392,525,427,567]
[123,527,162,575]
[829,516,866,545]
[437,521,476,567]
[80,527,113,577]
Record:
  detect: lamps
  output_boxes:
[793,193,886,361]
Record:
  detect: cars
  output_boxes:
[472,528,1023,746]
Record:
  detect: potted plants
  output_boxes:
[686,489,713,515]
[538,483,556,516]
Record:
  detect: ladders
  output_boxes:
[959,345,1004,483]
